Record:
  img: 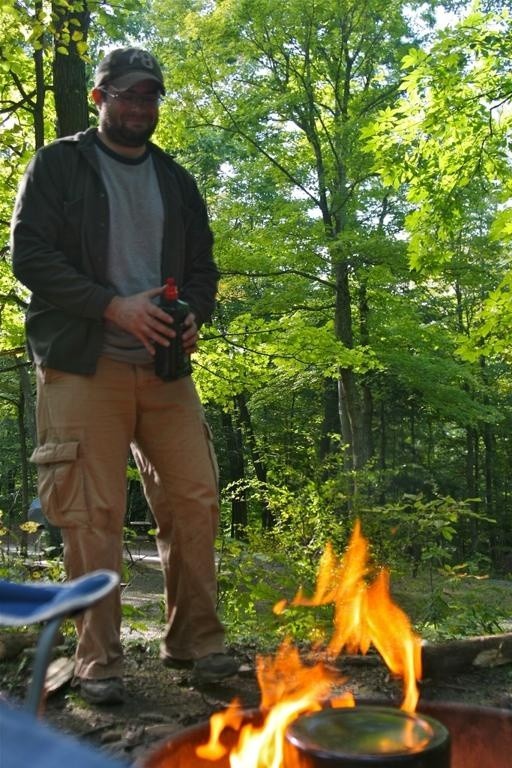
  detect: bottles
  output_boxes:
[155,277,194,381]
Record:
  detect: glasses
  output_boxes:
[96,87,168,107]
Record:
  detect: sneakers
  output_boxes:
[164,650,238,680]
[78,677,131,708]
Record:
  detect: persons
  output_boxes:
[11,47,250,708]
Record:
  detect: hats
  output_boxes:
[91,47,168,96]
